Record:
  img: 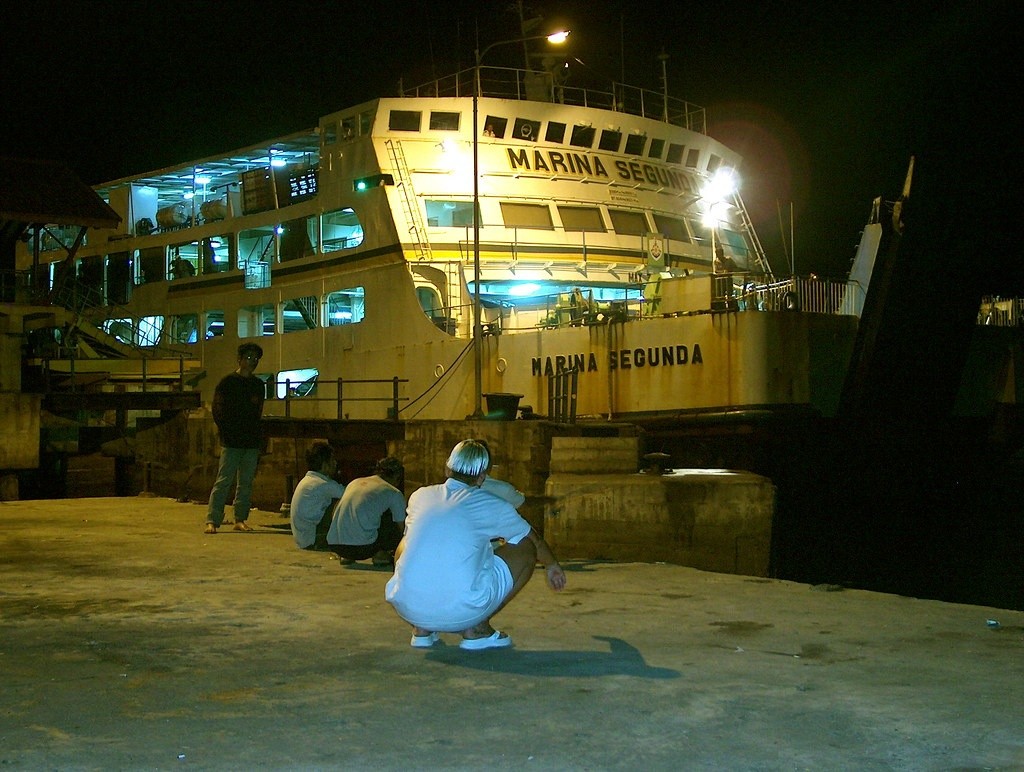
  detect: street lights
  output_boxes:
[470,23,576,420]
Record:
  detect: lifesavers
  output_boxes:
[777,290,801,311]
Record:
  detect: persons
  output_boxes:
[290,442,345,550]
[714,249,733,308]
[326,456,406,565]
[204,343,266,533]
[478,439,538,530]
[483,124,496,136]
[384,440,565,650]
[809,272,826,311]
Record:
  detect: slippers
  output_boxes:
[460,630,512,650]
[411,631,441,646]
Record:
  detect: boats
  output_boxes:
[2,0,920,444]
